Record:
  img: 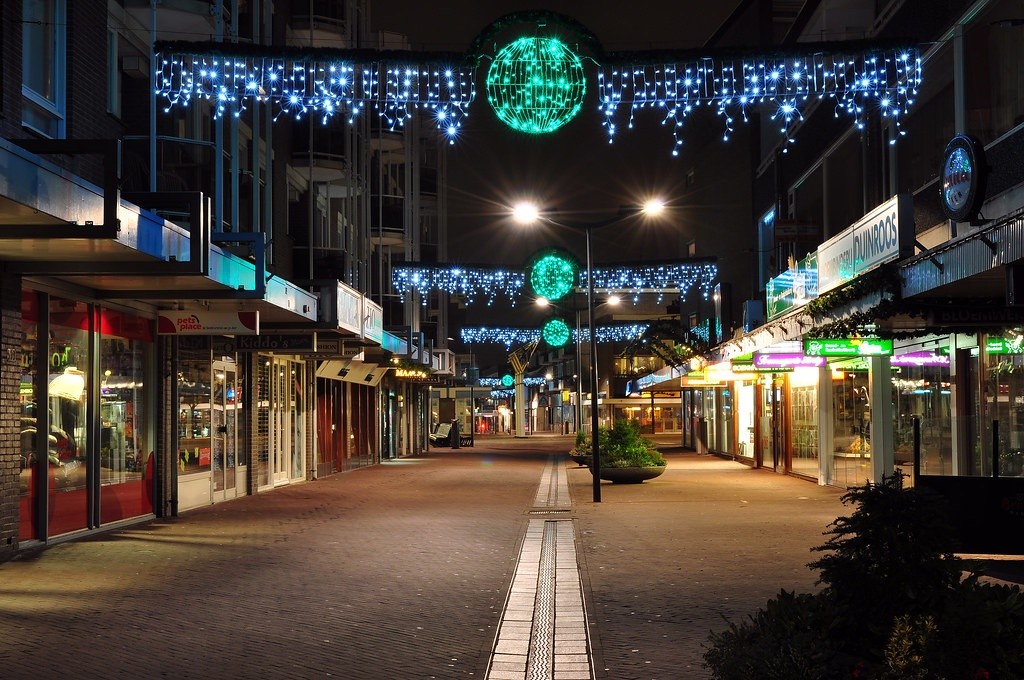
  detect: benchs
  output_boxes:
[429,422,472,447]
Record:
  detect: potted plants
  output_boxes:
[568,410,667,484]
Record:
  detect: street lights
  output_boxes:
[546,374,578,435]
[447,338,473,447]
[518,201,663,503]
[536,297,619,466]
